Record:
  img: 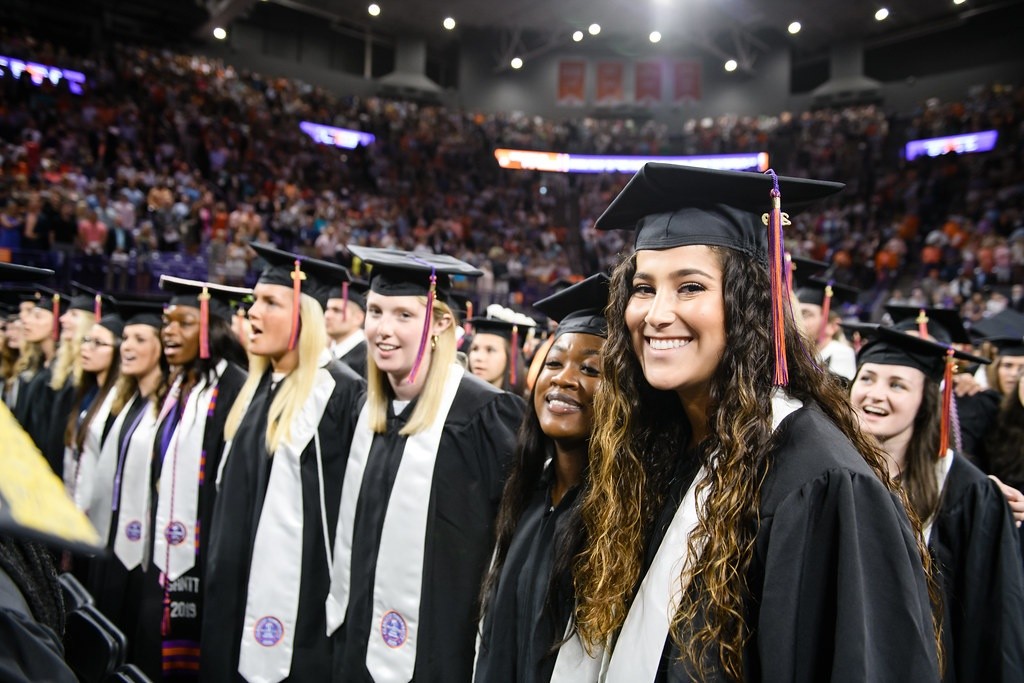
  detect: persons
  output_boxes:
[196,240,367,683]
[326,244,529,683]
[834,318,1024,683]
[0,263,540,683]
[770,257,1024,534]
[470,272,612,683]
[0,20,1024,324]
[547,160,944,683]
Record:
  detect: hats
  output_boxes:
[463,320,534,386]
[882,304,973,351]
[591,160,847,387]
[831,323,994,459]
[245,242,352,354]
[529,272,615,338]
[796,275,860,345]
[0,262,166,343]
[974,334,1023,356]
[348,241,485,386]
[329,275,371,322]
[157,273,256,361]
